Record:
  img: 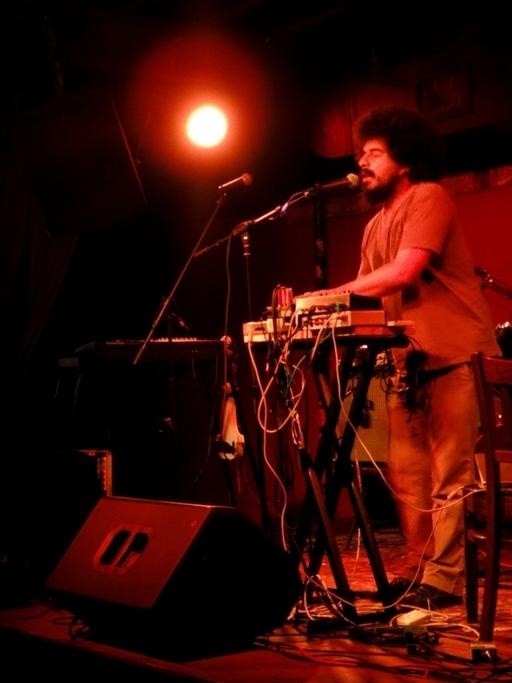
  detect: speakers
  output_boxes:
[43,495,302,648]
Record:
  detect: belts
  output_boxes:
[424,359,474,382]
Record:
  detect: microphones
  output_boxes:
[217,173,253,191]
[303,172,359,197]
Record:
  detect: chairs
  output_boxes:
[469,351,512,642]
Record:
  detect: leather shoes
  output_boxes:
[370,575,422,603]
[397,581,465,609]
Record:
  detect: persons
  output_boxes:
[289,106,490,609]
